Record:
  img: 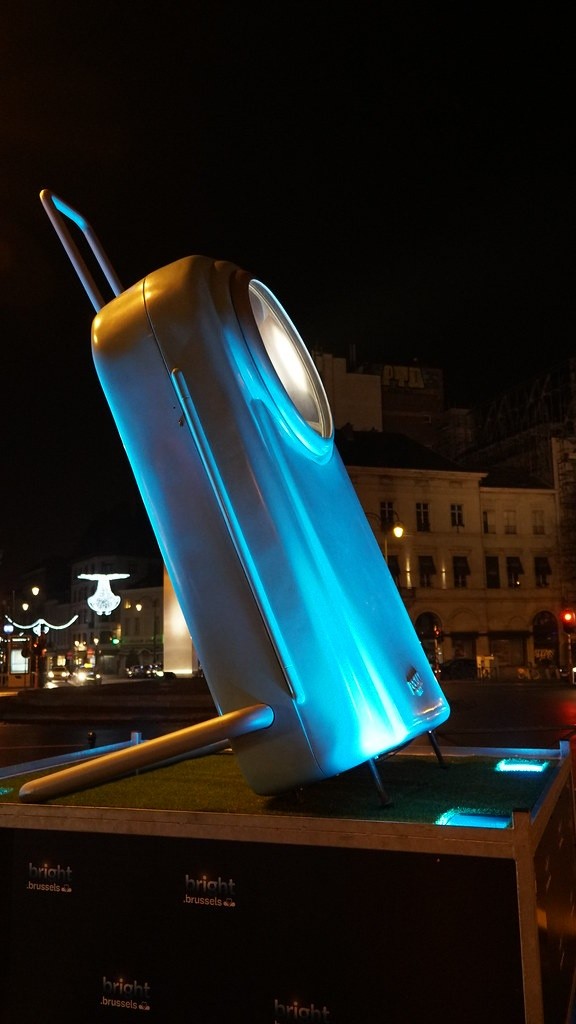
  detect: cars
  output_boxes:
[128,664,162,679]
[439,658,476,679]
[74,668,102,685]
[48,667,69,682]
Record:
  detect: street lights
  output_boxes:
[366,510,404,566]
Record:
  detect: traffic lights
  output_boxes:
[563,608,576,633]
